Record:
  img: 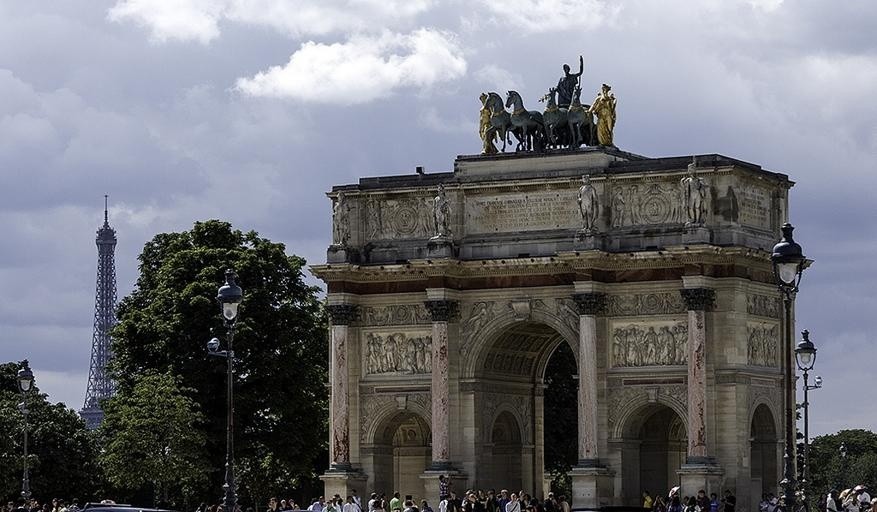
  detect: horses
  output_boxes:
[565,85,595,149]
[542,87,569,152]
[482,92,524,153]
[503,89,544,153]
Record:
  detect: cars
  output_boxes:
[77,502,180,512]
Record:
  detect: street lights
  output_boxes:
[794,327,822,512]
[206,270,244,512]
[17,359,35,512]
[770,222,807,512]
[839,442,847,490]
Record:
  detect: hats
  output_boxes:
[548,492,554,496]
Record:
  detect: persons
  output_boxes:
[586,84,617,142]
[817,488,877,511]
[439,476,450,502]
[679,163,707,227]
[609,187,626,230]
[576,174,598,233]
[331,191,350,247]
[198,498,224,512]
[2,498,80,511]
[431,184,452,238]
[643,489,736,512]
[307,487,433,512]
[556,55,584,105]
[759,489,808,511]
[625,185,642,225]
[438,488,570,511]
[654,182,681,225]
[267,497,300,511]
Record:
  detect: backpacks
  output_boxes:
[544,497,554,512]
[472,500,485,512]
